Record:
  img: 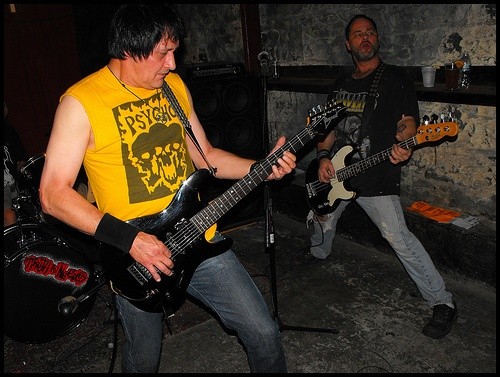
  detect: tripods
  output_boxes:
[263,81,340,334]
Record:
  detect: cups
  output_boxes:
[445,63,460,91]
[421,66,436,88]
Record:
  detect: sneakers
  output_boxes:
[297,249,330,263]
[421,298,456,339]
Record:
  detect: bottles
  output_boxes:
[458,52,471,89]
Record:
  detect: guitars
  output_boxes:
[101,98,346,313]
[303,109,459,216]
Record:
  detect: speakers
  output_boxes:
[189,72,269,232]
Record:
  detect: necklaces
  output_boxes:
[108,66,166,125]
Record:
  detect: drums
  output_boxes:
[0,220,99,345]
[11,191,42,219]
[18,152,80,198]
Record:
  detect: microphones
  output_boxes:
[258,50,270,79]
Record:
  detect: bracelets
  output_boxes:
[248,160,260,171]
[317,148,330,159]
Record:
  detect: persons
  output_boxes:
[4,102,28,228]
[39,0,299,377]
[293,14,458,337]
